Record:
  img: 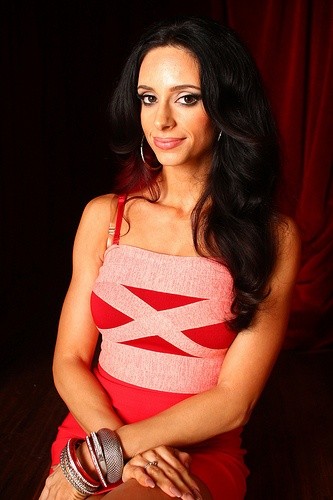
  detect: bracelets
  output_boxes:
[58,426,124,496]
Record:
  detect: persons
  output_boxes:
[36,17,298,498]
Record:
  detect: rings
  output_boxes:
[144,461,158,468]
